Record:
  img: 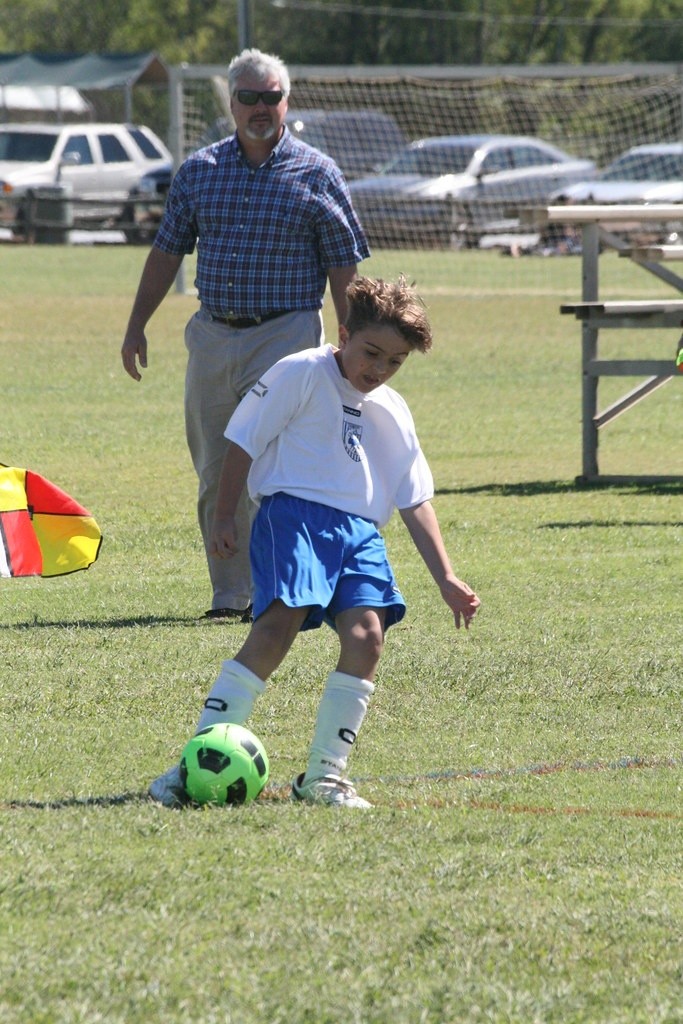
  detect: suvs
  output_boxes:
[123,104,403,244]
[0,122,172,229]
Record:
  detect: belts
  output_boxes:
[209,307,296,328]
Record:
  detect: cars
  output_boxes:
[553,141,683,237]
[350,134,601,254]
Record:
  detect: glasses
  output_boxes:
[235,89,284,106]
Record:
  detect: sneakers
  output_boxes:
[289,771,373,810]
[147,764,191,811]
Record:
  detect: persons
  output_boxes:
[122,47,373,627]
[503,193,604,259]
[148,276,480,810]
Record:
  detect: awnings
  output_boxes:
[0,49,169,91]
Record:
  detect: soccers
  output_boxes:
[177,718,272,809]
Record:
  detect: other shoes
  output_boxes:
[209,604,255,624]
